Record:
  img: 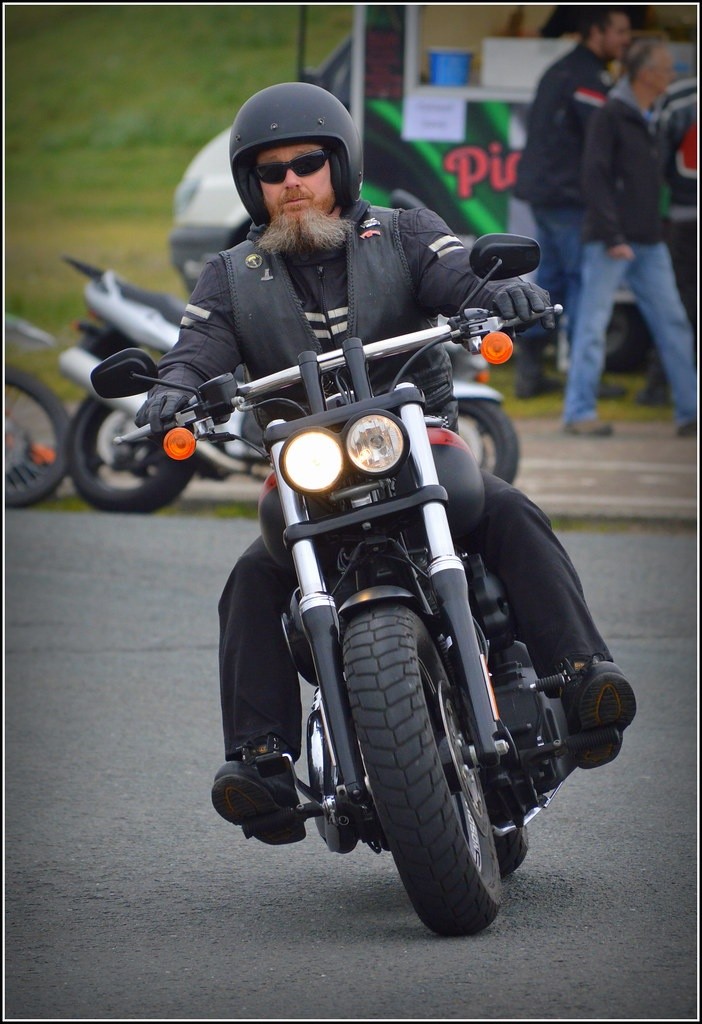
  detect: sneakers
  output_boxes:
[211,734,306,845]
[558,652,637,769]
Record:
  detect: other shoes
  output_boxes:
[676,420,696,436]
[564,419,611,436]
[515,378,563,399]
[599,384,626,398]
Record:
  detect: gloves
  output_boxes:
[134,390,195,448]
[488,281,556,342]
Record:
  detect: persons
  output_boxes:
[124,80,636,845]
[516,7,630,401]
[559,32,698,442]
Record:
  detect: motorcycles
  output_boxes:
[5,307,73,510]
[51,255,524,516]
[92,232,623,935]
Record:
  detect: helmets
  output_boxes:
[228,81,364,226]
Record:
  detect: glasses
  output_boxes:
[255,147,330,184]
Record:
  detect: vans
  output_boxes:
[169,33,360,288]
